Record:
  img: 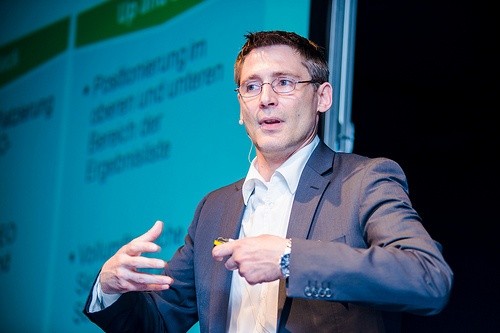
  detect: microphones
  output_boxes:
[239,110,244,125]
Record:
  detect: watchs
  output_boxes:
[279,238,291,280]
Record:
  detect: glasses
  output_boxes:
[234,76,321,98]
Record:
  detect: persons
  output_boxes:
[82,30,454,333]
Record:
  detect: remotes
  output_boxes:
[214,239,228,246]
